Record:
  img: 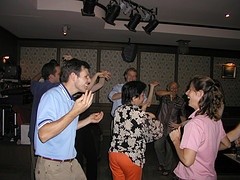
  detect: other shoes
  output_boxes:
[159,165,163,170]
[162,170,170,175]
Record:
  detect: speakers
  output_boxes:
[121,43,137,62]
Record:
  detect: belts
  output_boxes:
[39,156,74,162]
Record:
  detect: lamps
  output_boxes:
[142,12,159,37]
[176,39,192,54]
[120,37,137,62]
[81,0,98,18]
[101,0,121,26]
[62,25,70,36]
[124,7,142,33]
[220,61,237,80]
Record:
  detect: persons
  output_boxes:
[34,60,104,180]
[169,75,231,180]
[155,82,186,176]
[28,59,110,180]
[108,68,158,116]
[109,80,163,180]
